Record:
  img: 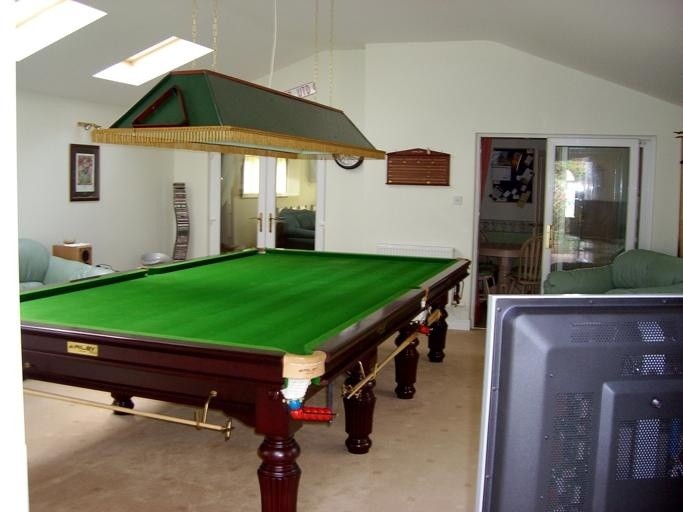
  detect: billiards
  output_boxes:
[289,400,333,421]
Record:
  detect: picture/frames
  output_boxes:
[70,143,101,202]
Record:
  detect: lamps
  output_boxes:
[94,2,384,166]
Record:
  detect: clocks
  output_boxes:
[333,150,364,169]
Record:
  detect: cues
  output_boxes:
[23,388,231,441]
[341,309,440,399]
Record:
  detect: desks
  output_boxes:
[19,246,470,511]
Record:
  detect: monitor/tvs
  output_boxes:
[574,200,626,242]
[475,291,683,511]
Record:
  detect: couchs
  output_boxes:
[19,237,116,297]
[275,208,314,250]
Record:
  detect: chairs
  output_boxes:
[545,249,683,295]
[479,227,541,301]
